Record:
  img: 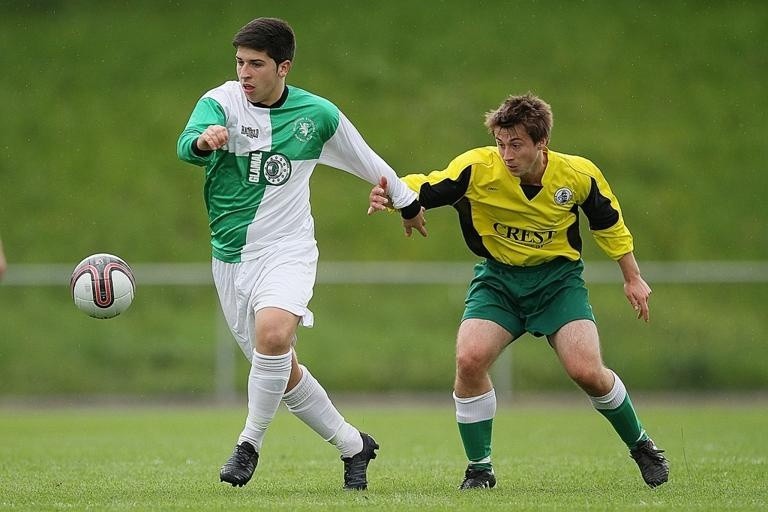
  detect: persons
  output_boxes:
[173,14,429,497]
[364,89,672,492]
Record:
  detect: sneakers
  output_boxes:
[459,462,497,491]
[219,442,259,487]
[630,438,669,489]
[340,431,379,489]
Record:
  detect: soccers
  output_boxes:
[69,253,136,320]
